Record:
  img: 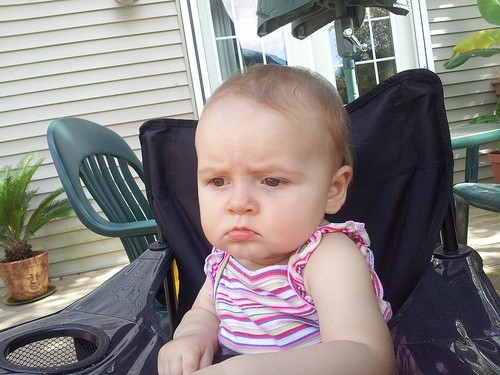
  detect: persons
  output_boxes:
[158,62,449,375]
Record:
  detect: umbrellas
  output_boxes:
[255,0,411,105]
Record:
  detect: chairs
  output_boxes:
[452,129,500,246]
[46,117,158,263]
[0,69,499,375]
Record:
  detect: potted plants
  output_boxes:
[0,151,73,305]
[469,95,500,185]
[443,0,500,97]
